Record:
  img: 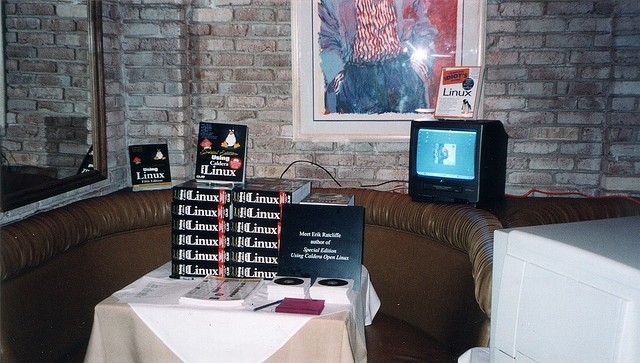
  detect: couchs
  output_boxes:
[506,195,640,231]
[1,175,503,363]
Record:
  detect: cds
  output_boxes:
[318,278,348,287]
[274,278,304,285]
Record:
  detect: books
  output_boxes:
[434,65,486,119]
[169,178,365,281]
[128,143,171,191]
[274,297,325,316]
[195,121,248,184]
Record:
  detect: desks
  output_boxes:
[85,259,380,363]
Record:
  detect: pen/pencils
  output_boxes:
[254,300,281,310]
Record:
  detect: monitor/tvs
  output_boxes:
[408,119,508,208]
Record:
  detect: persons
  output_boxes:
[317,0,440,115]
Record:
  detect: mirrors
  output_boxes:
[3,2,109,212]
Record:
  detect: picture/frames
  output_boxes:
[290,0,488,146]
